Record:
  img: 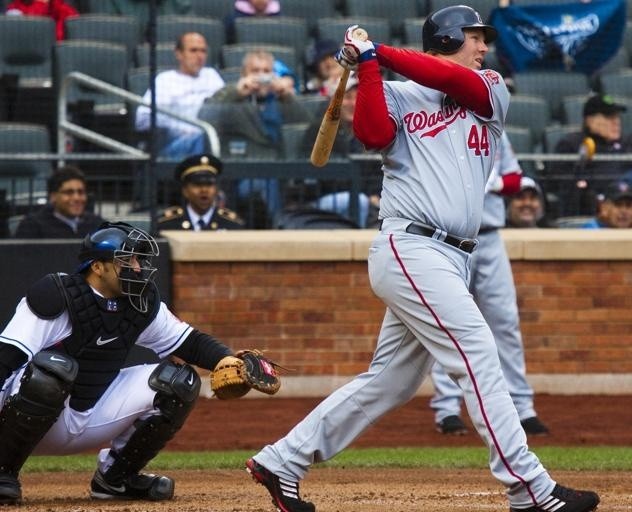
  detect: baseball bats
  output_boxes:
[310,27,368,168]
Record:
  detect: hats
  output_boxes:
[173,152,223,184]
[604,181,632,201]
[504,177,544,208]
[582,93,626,114]
[304,38,338,73]
[329,71,360,98]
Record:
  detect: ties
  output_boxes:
[198,219,206,230]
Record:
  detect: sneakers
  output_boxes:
[245,457,315,512]
[90,468,174,501]
[520,417,550,437]
[510,484,600,512]
[435,415,470,435]
[0,475,22,505]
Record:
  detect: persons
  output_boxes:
[0,229,280,507]
[246,5,600,512]
[428,130,551,438]
[0,1,630,239]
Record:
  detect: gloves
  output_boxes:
[335,24,379,69]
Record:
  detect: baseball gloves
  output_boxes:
[211,348,281,400]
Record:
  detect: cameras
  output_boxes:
[256,75,275,86]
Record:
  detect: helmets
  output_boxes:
[74,221,160,313]
[422,5,498,55]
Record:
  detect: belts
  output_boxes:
[379,217,476,254]
